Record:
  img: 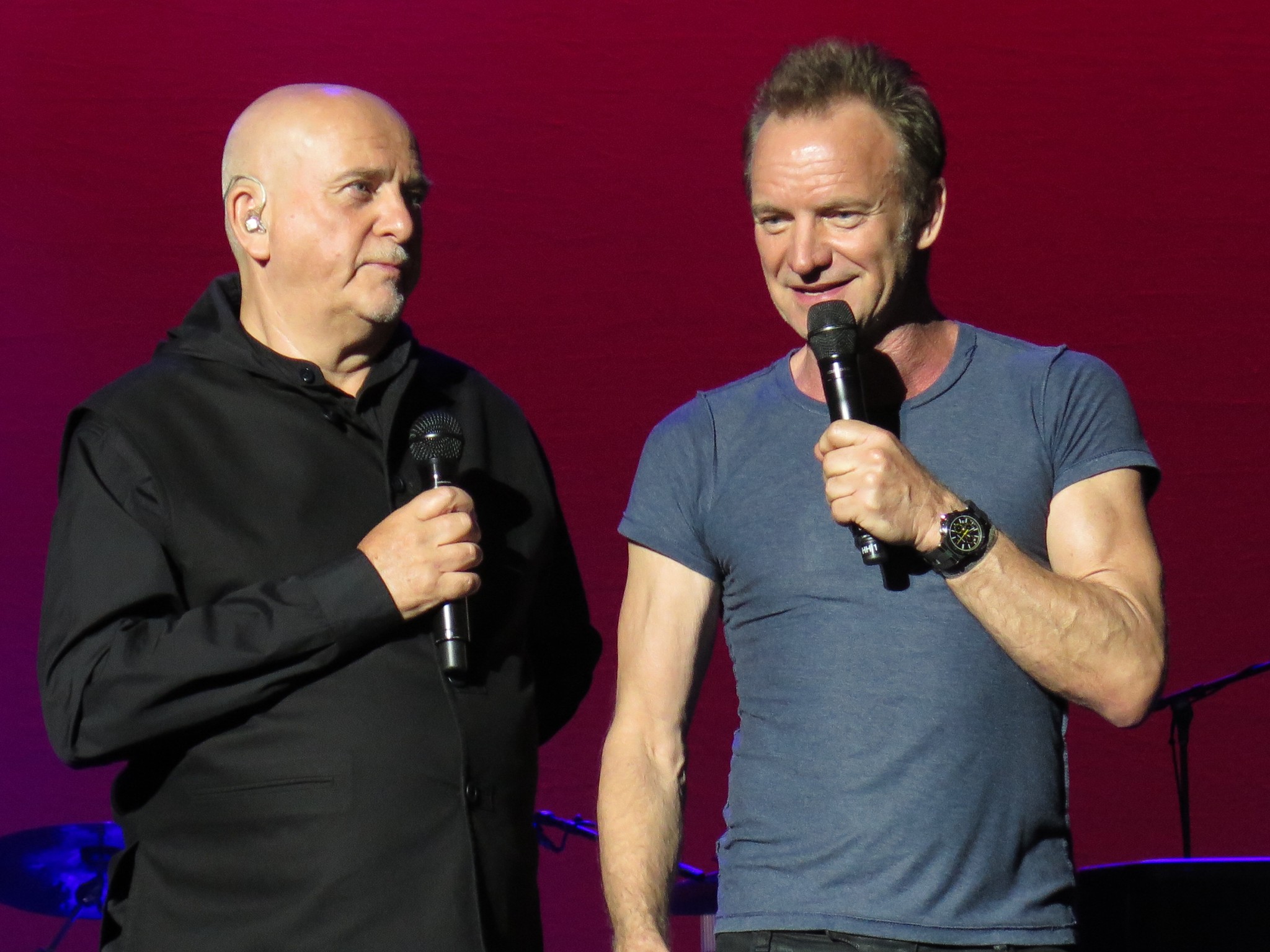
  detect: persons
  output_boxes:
[593,39,1176,952]
[34,80,602,952]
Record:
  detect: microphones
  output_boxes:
[408,411,470,686]
[806,300,890,565]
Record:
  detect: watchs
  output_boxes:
[922,497,989,576]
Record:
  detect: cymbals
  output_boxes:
[0,821,127,918]
